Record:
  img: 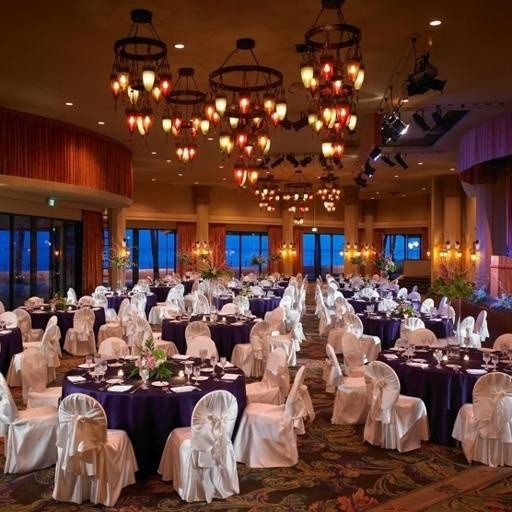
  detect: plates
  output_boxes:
[78,363,96,368]
[108,364,123,367]
[67,376,86,382]
[466,369,487,374]
[481,365,498,368]
[89,371,106,376]
[200,368,213,372]
[107,385,133,392]
[217,361,234,368]
[446,364,462,368]
[390,347,405,351]
[407,363,429,368]
[191,376,208,380]
[415,350,428,352]
[221,374,238,380]
[120,355,139,360]
[384,354,398,360]
[0,331,12,334]
[180,361,194,364]
[151,381,169,387]
[413,358,428,363]
[172,354,192,360]
[170,386,196,393]
[106,379,124,383]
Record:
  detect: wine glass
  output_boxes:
[94,365,102,383]
[139,366,150,390]
[464,337,470,352]
[122,345,129,364]
[115,348,121,364]
[210,356,217,375]
[99,361,107,381]
[193,366,200,385]
[185,363,192,385]
[483,353,491,370]
[220,358,226,374]
[117,369,124,385]
[492,356,498,371]
[367,304,374,316]
[86,356,93,373]
[94,353,101,362]
[434,350,443,368]
[0,318,5,330]
[395,338,415,363]
[200,349,208,367]
